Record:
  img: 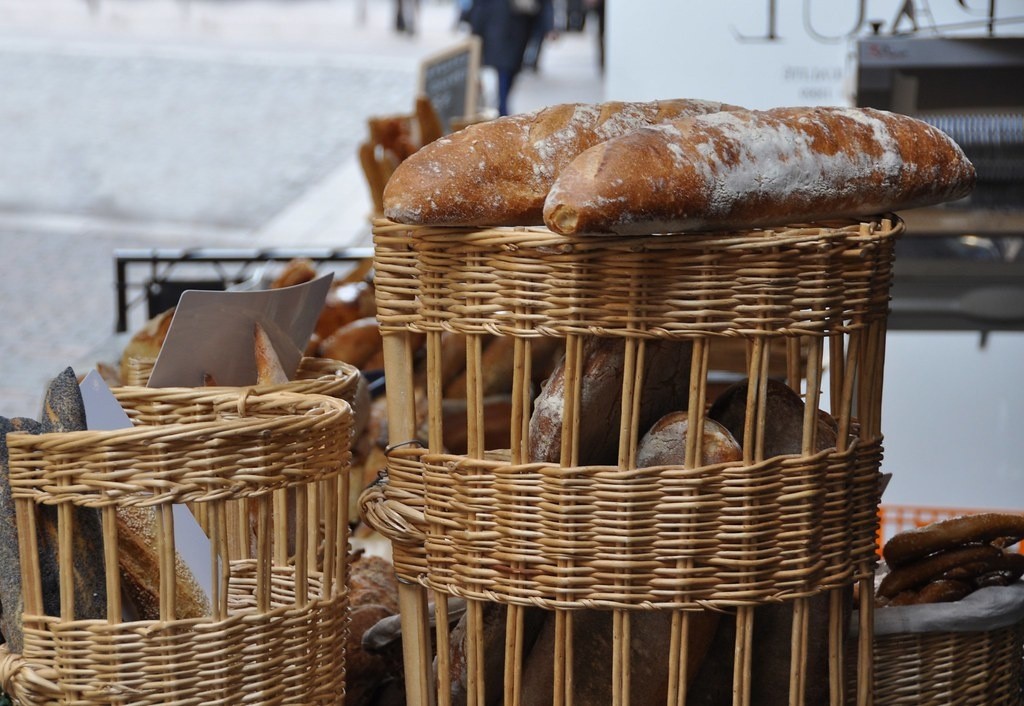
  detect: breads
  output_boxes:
[0,94,1024,706]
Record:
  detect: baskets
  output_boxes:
[72,353,360,411]
[876,505,1024,558]
[846,585,1024,706]
[0,387,354,706]
[359,216,902,704]
